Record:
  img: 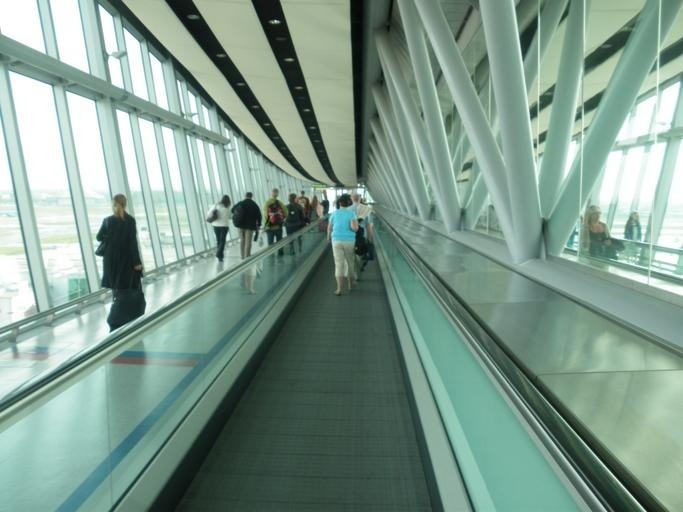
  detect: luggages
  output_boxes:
[107,289,146,333]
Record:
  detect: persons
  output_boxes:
[210,188,373,282]
[580,206,612,258]
[327,193,359,295]
[241,263,260,294]
[567,213,585,249]
[97,301,143,439]
[623,211,641,264]
[482,205,499,230]
[96,194,141,298]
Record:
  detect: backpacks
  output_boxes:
[286,207,303,233]
[310,207,318,221]
[268,200,284,225]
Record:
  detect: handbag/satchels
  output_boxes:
[206,208,218,223]
[95,240,107,256]
[363,239,375,261]
